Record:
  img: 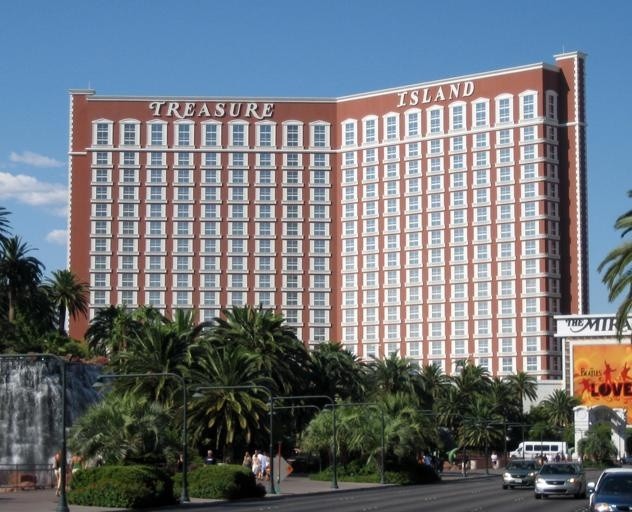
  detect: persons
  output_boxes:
[49,449,62,496]
[70,450,84,473]
[205,449,217,465]
[243,450,270,480]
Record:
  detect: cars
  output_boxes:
[502,459,632,512]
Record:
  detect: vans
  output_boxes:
[510,441,570,462]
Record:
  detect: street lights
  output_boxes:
[268,405,321,416]
[475,420,524,474]
[91,373,188,501]
[265,395,337,488]
[192,386,275,494]
[321,403,385,484]
